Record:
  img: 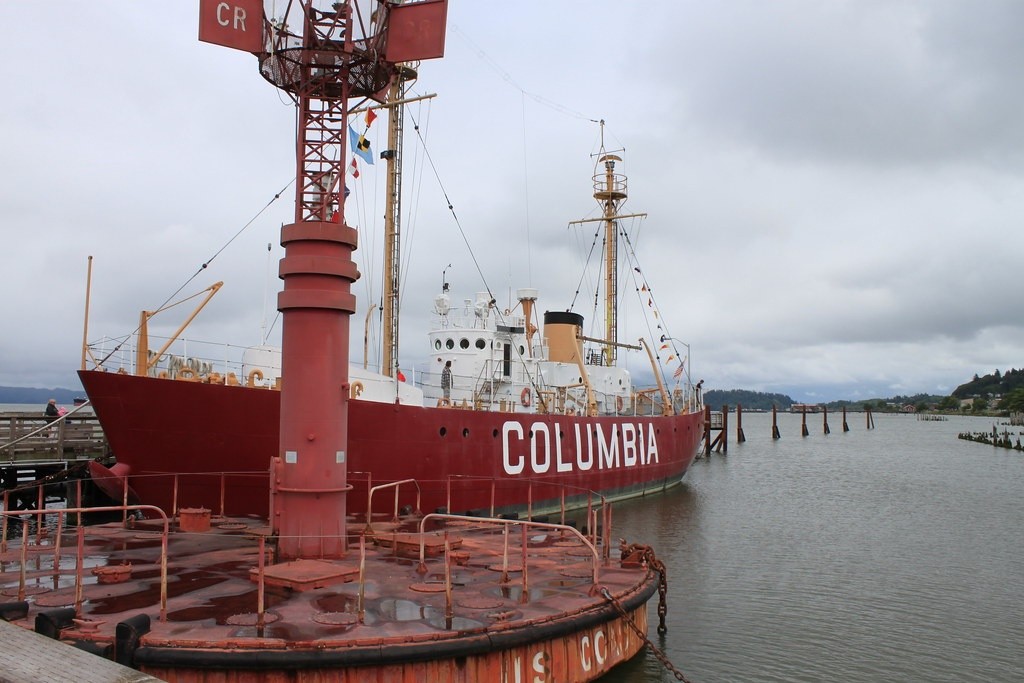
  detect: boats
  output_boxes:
[70,106,711,525]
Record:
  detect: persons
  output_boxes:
[43,399,62,425]
[442,361,453,406]
[696,380,704,405]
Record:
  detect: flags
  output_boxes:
[673,360,685,379]
[344,184,349,203]
[330,210,346,225]
[397,369,406,383]
[356,134,370,153]
[364,107,377,127]
[349,124,374,166]
[634,266,674,365]
[349,157,359,179]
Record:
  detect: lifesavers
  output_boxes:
[614,396,623,411]
[521,387,533,408]
